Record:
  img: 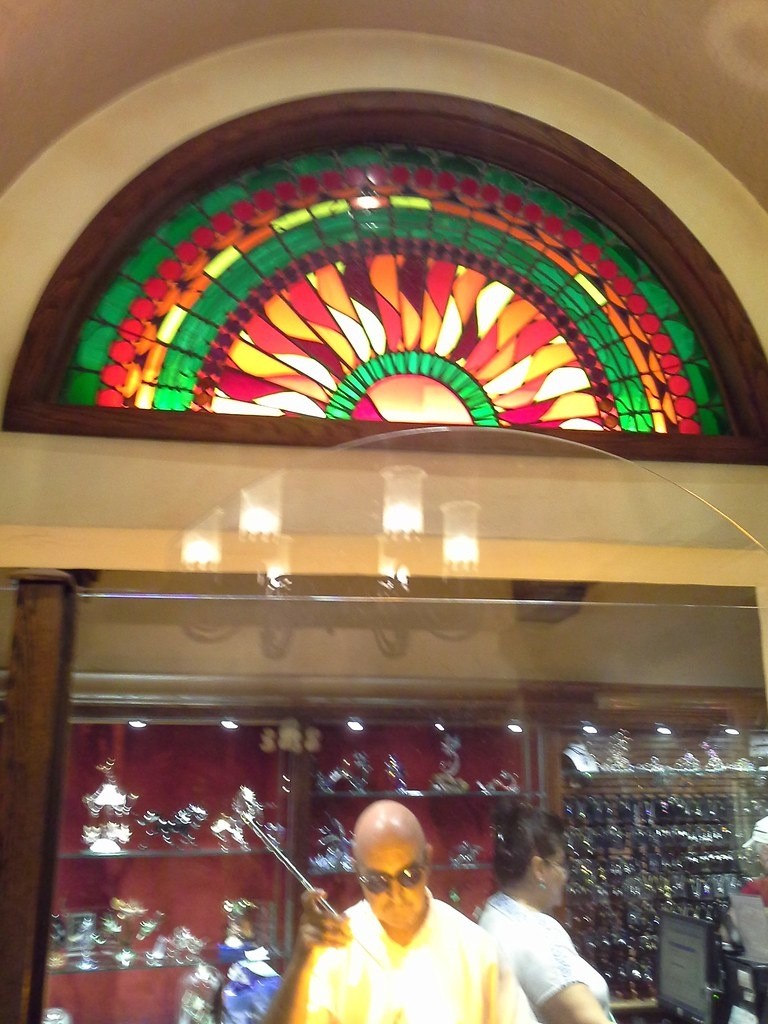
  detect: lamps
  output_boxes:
[177,460,486,659]
[357,186,382,209]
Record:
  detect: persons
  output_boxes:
[257,799,540,1023]
[478,806,618,1024]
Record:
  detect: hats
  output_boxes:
[742,817,768,848]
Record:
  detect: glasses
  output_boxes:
[355,858,424,894]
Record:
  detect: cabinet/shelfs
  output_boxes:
[38,717,759,1023]
